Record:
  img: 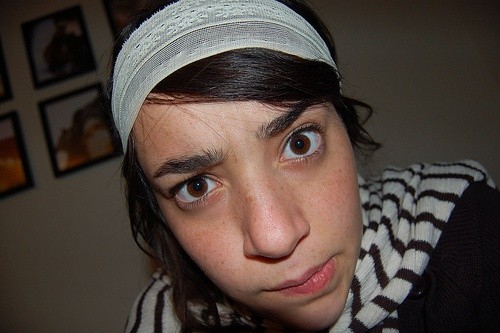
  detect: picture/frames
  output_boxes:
[38,82,124,178]
[20,4,99,90]
[0,111,35,201]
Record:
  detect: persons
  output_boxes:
[99,0,500,333]
